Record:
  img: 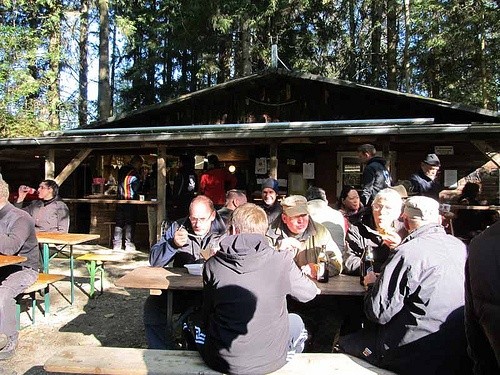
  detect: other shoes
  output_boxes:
[113,245,140,254]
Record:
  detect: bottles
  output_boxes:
[360,244,372,287]
[23,187,38,194]
[317,243,328,282]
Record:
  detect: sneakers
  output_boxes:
[0,337,20,360]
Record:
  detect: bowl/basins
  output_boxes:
[183,264,203,275]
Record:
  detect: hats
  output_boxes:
[306,187,327,202]
[422,153,440,168]
[261,178,279,193]
[401,195,442,225]
[280,195,310,217]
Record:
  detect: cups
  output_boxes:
[140,195,145,201]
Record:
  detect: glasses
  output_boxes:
[189,211,213,222]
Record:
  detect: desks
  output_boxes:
[439,204,500,237]
[36,232,100,305]
[114,265,366,350]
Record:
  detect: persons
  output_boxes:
[1,143,499,375]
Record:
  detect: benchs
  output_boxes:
[43,346,399,375]
[49,254,123,299]
[22,273,66,325]
[104,221,149,246]
